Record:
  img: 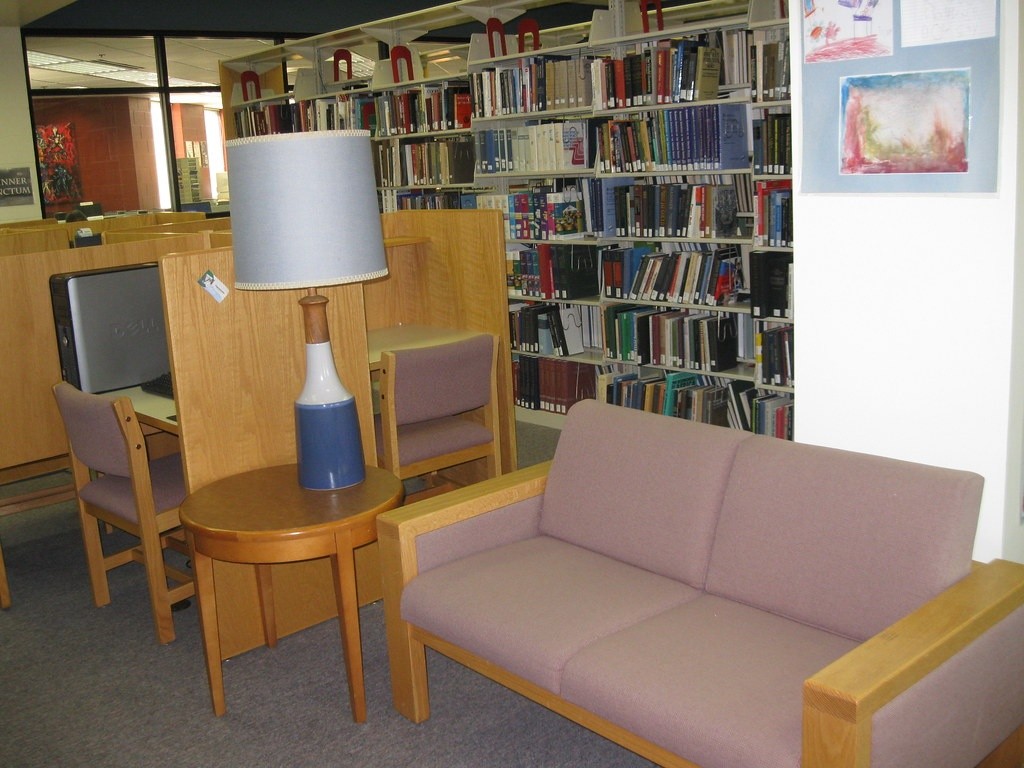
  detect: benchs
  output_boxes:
[374,400,1024,768]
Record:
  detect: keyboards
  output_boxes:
[141,370,174,401]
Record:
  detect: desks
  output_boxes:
[369,322,490,373]
[183,463,405,722]
[98,387,181,436]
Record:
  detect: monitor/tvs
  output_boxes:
[181,202,212,216]
[77,203,103,216]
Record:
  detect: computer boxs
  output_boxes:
[49,261,170,395]
[69,233,102,248]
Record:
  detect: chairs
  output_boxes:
[375,332,500,502]
[53,381,196,646]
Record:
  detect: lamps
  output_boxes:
[224,129,388,491]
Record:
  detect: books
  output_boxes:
[474,120,589,173]
[598,364,794,440]
[508,299,603,358]
[512,354,603,415]
[590,26,791,102]
[372,133,476,186]
[602,242,794,319]
[592,174,793,249]
[378,190,478,213]
[234,101,291,138]
[505,243,598,300]
[289,82,471,138]
[603,303,794,388]
[468,53,594,117]
[586,103,791,174]
[475,177,586,241]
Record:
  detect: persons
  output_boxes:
[66,210,89,222]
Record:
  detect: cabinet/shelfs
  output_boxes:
[219,0,794,440]
[175,157,201,203]
